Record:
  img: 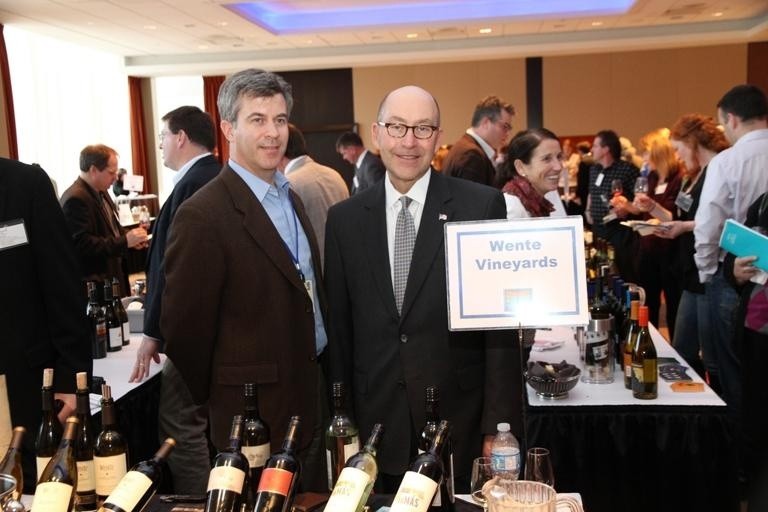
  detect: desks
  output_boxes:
[525,324,727,512]
[85,331,167,464]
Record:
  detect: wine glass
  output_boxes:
[525,447,554,488]
[471,457,495,512]
[634,176,649,197]
[139,212,151,231]
[611,179,623,203]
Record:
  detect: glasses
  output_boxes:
[377,122,439,140]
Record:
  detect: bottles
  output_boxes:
[389,421,449,512]
[581,261,659,401]
[30,416,81,512]
[203,413,250,512]
[489,422,521,482]
[32,368,58,483]
[320,422,384,512]
[96,438,174,512]
[246,414,299,512]
[103,285,122,352]
[415,387,442,511]
[239,381,270,503]
[85,287,106,360]
[73,370,96,508]
[324,381,360,496]
[92,384,127,505]
[111,281,130,347]
[0,425,25,512]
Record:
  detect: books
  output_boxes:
[657,356,681,365]
[719,218,767,274]
[659,361,688,373]
[620,220,674,236]
[659,370,693,383]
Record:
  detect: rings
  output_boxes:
[139,364,145,366]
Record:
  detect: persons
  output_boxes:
[160,68,330,502]
[722,190,768,512]
[0,155,93,495]
[113,168,130,197]
[336,131,386,196]
[496,144,514,188]
[60,143,148,301]
[493,127,563,217]
[693,84,768,512]
[324,84,523,494]
[441,95,515,186]
[584,129,640,282]
[278,121,349,278]
[558,139,581,195]
[639,127,685,346]
[632,112,731,383]
[618,136,644,171]
[571,141,596,210]
[128,106,222,496]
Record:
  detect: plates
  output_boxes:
[524,365,581,400]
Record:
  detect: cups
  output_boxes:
[485,481,582,512]
[132,207,139,221]
[0,474,24,512]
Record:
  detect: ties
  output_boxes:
[394,196,416,318]
